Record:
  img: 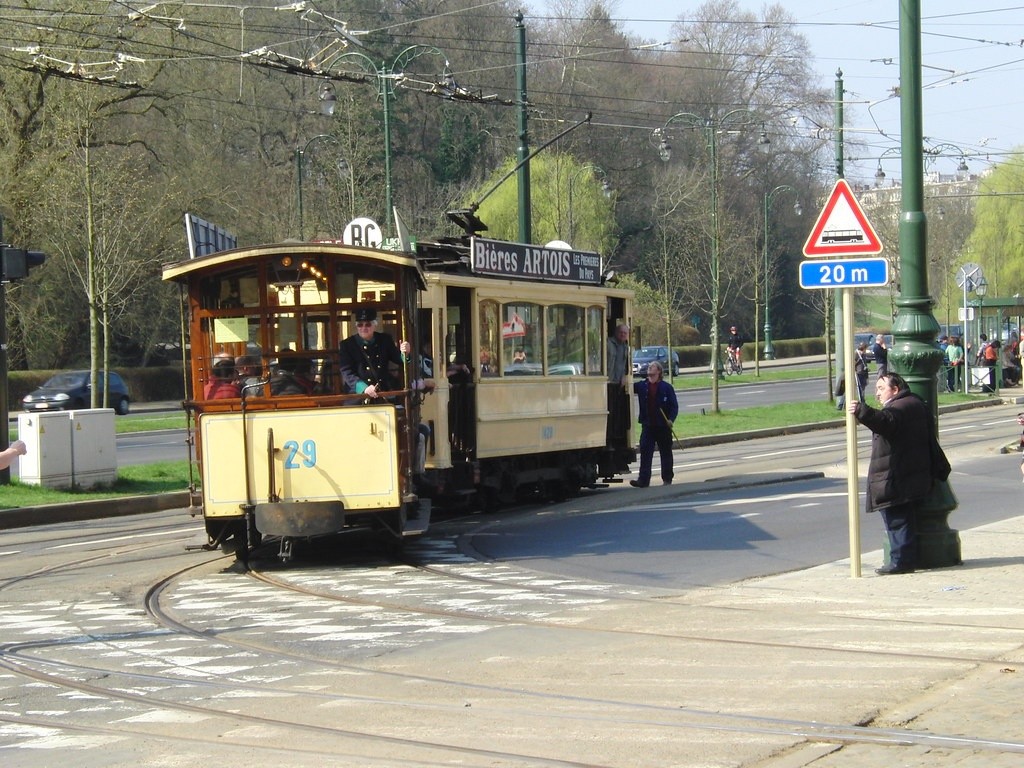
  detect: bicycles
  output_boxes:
[723,349,744,375]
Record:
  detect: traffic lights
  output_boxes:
[1,244,47,282]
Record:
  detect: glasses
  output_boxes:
[357,323,372,327]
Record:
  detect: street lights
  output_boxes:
[764,184,802,362]
[315,41,451,243]
[876,141,969,180]
[654,107,768,410]
[568,162,612,252]
[921,196,946,220]
[976,275,986,394]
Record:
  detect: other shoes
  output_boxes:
[630,480,649,488]
[1010,383,1016,386]
[662,480,672,486]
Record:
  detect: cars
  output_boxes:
[936,323,1018,350]
[23,368,129,418]
[853,332,894,363]
[633,346,681,379]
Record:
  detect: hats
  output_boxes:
[355,308,376,322]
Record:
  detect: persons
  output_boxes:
[629,361,679,488]
[202,346,352,402]
[0,440,28,471]
[848,371,934,576]
[835,334,888,411]
[726,326,744,367]
[1015,413,1024,484]
[338,306,411,406]
[410,329,500,484]
[607,324,634,471]
[936,332,1024,393]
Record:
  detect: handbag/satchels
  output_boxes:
[930,433,951,481]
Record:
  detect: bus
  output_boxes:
[156,235,635,572]
